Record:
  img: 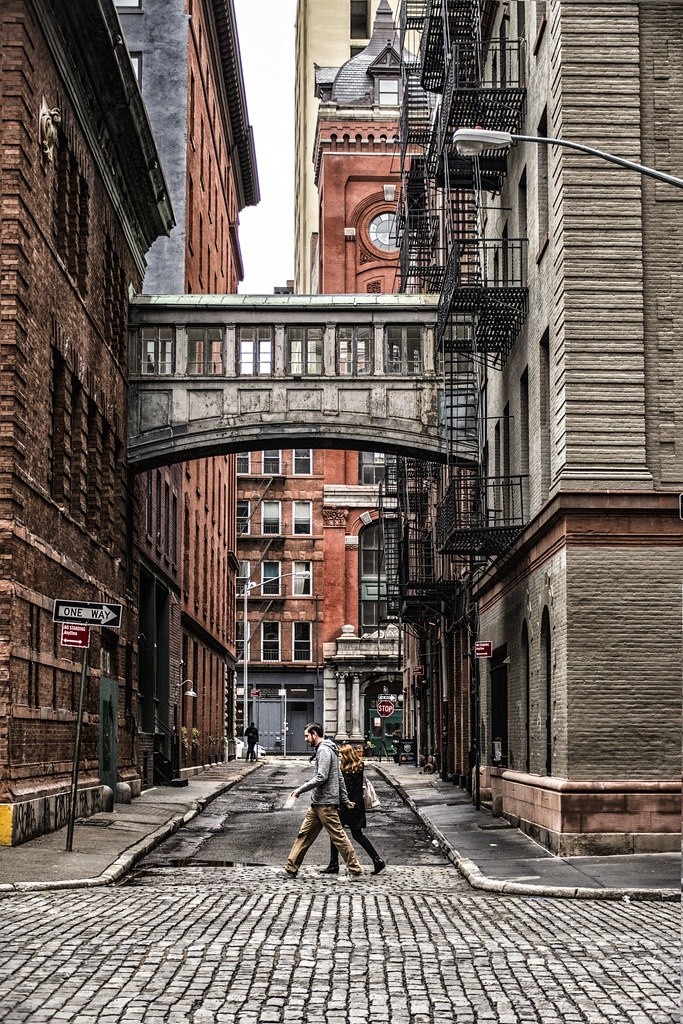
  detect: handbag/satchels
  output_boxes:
[362,770,380,809]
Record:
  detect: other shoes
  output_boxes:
[271,868,296,879]
[337,874,367,882]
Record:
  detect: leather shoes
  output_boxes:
[370,856,385,875]
[319,862,339,873]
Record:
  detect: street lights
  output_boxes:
[243,570,311,736]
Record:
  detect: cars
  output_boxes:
[235,736,266,758]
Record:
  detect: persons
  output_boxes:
[244,721,259,763]
[275,723,367,882]
[319,744,386,874]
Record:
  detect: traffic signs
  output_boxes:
[52,599,122,628]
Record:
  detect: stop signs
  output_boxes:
[376,700,395,718]
